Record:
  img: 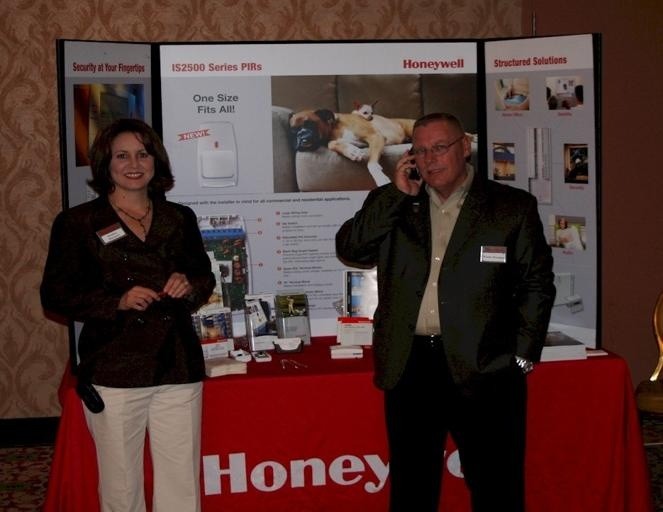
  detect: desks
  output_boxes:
[45,337,651,510]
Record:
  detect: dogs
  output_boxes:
[351,99,379,121]
[287,108,478,187]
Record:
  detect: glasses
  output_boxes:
[413,136,462,160]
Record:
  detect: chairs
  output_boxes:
[635,288,661,445]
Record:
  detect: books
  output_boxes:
[189,293,311,378]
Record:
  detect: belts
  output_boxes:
[413,333,442,347]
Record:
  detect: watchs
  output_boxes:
[514,356,535,373]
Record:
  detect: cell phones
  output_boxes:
[408,151,420,180]
[139,289,170,306]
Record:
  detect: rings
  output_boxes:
[184,280,189,288]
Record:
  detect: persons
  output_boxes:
[334,112,556,512]
[40,118,217,512]
[557,218,574,248]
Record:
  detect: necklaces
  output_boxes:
[108,195,151,237]
